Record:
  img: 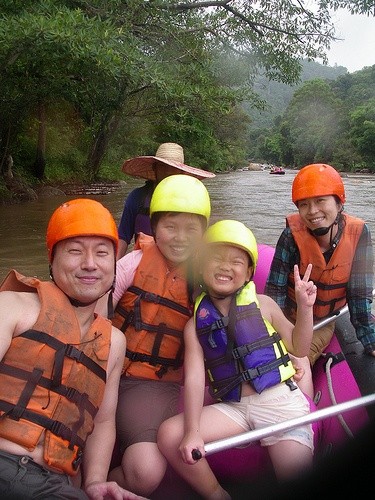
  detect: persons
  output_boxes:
[263,163,375,367]
[94,174,305,500]
[0,198,149,500]
[271,165,283,172]
[156,219,317,500]
[118,143,216,258]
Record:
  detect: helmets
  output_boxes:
[203,219,259,275]
[149,173,212,228]
[44,198,120,265]
[292,164,346,207]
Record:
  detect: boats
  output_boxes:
[269,170,286,175]
[102,243,372,485]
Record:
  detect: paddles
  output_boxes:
[192,392,375,460]
[313,287,375,331]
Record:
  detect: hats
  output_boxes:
[121,142,217,181]
